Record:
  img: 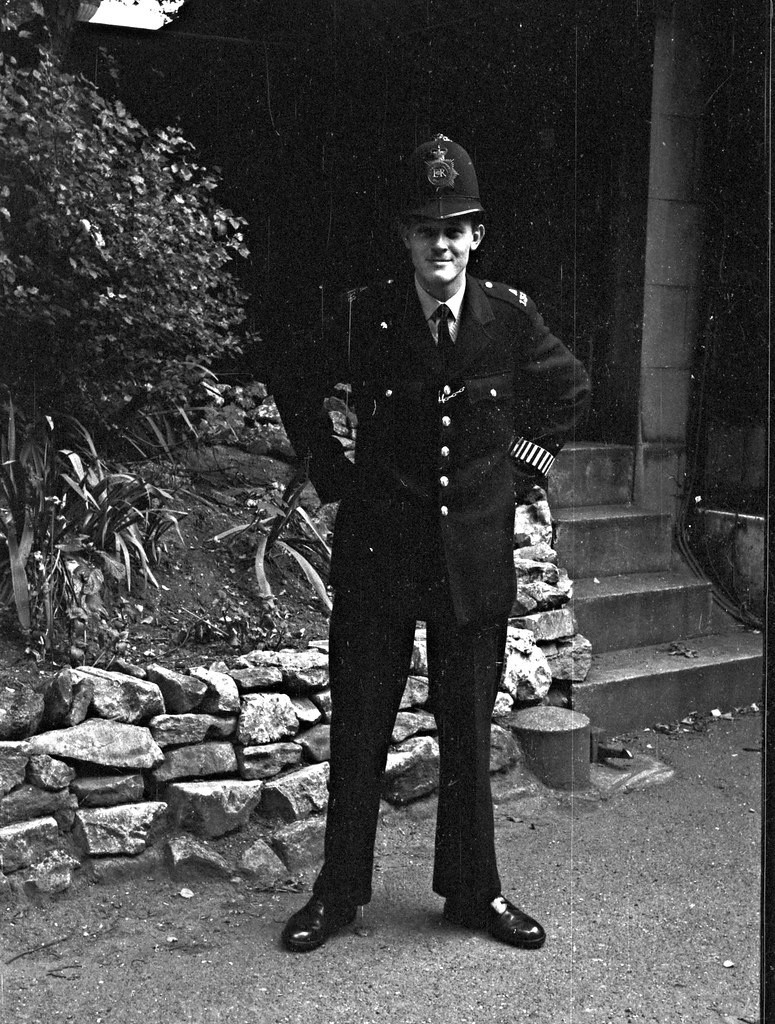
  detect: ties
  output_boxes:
[433,304,455,369]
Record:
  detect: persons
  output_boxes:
[265,133,592,951]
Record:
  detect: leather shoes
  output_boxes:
[282,893,358,953]
[443,892,546,950]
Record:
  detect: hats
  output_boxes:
[398,133,485,227]
